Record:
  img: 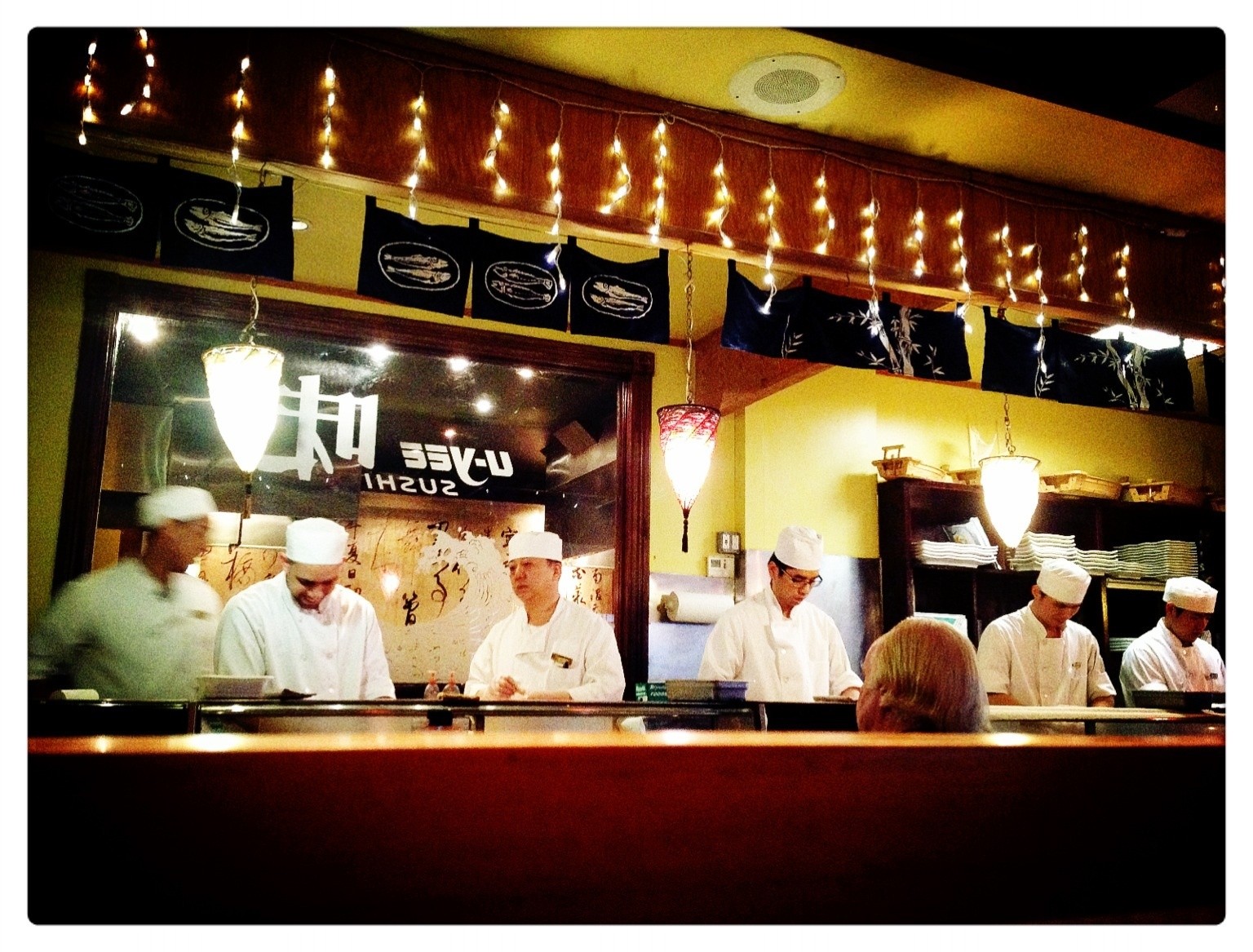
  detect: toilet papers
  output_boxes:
[666,592,733,624]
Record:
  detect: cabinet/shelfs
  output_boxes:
[879,476,1224,699]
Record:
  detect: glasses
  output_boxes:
[782,570,822,587]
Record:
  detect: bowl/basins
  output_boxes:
[1131,690,1224,714]
[196,675,275,698]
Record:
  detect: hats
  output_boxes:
[1036,558,1091,603]
[137,485,212,527]
[1162,577,1218,613]
[508,531,562,562]
[774,526,823,570]
[285,517,349,565]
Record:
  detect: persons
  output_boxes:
[464,532,625,736]
[698,528,863,701]
[28,486,221,701]
[856,617,990,734]
[977,558,1116,734]
[214,519,395,733]
[1119,577,1226,706]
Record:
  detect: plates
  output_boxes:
[1109,637,1138,651]
[912,540,999,568]
[1005,530,1199,581]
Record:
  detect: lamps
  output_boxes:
[202,272,286,520]
[662,112,721,555]
[983,297,1044,554]
